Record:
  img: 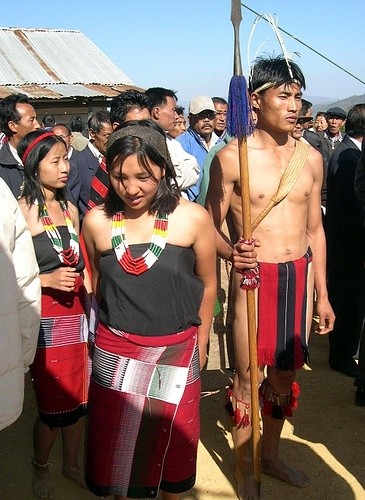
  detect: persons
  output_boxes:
[0,58,365,500]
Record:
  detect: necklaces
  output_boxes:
[110,210,169,276]
[33,198,79,267]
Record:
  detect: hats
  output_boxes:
[325,107,346,118]
[188,95,218,115]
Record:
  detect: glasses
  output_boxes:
[297,117,313,124]
[59,134,71,139]
[192,111,217,120]
